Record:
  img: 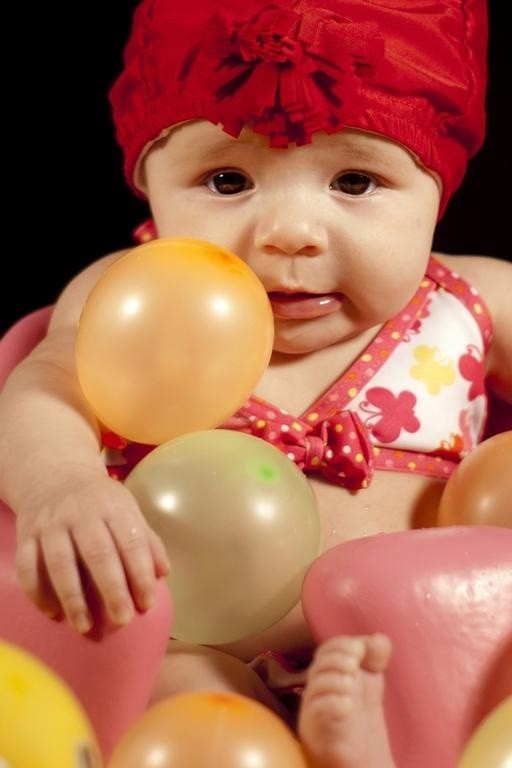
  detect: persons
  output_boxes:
[0,0,511,768]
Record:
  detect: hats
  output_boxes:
[108,0,489,222]
